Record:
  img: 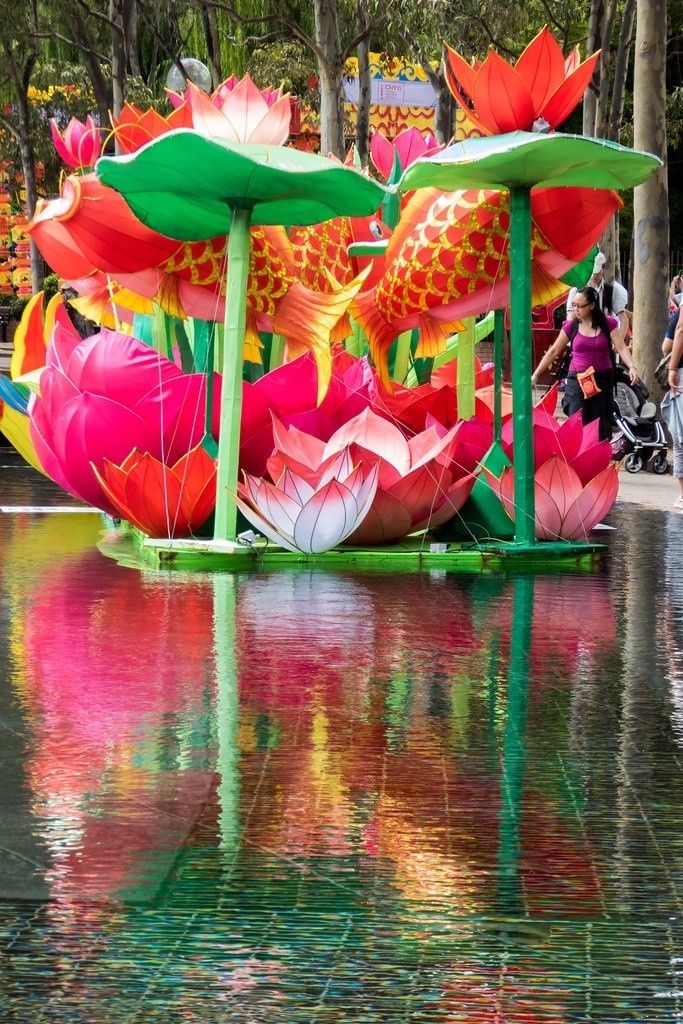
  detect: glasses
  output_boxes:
[571,301,592,309]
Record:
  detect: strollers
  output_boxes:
[557,350,669,475]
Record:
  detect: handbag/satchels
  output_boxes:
[575,366,600,399]
[652,351,671,393]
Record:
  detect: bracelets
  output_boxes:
[629,365,637,369]
[668,367,678,370]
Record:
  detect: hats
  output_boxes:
[593,250,607,274]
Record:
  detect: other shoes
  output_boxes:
[672,495,683,509]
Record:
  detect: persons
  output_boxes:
[565,253,629,366]
[662,265,683,510]
[531,286,640,443]
